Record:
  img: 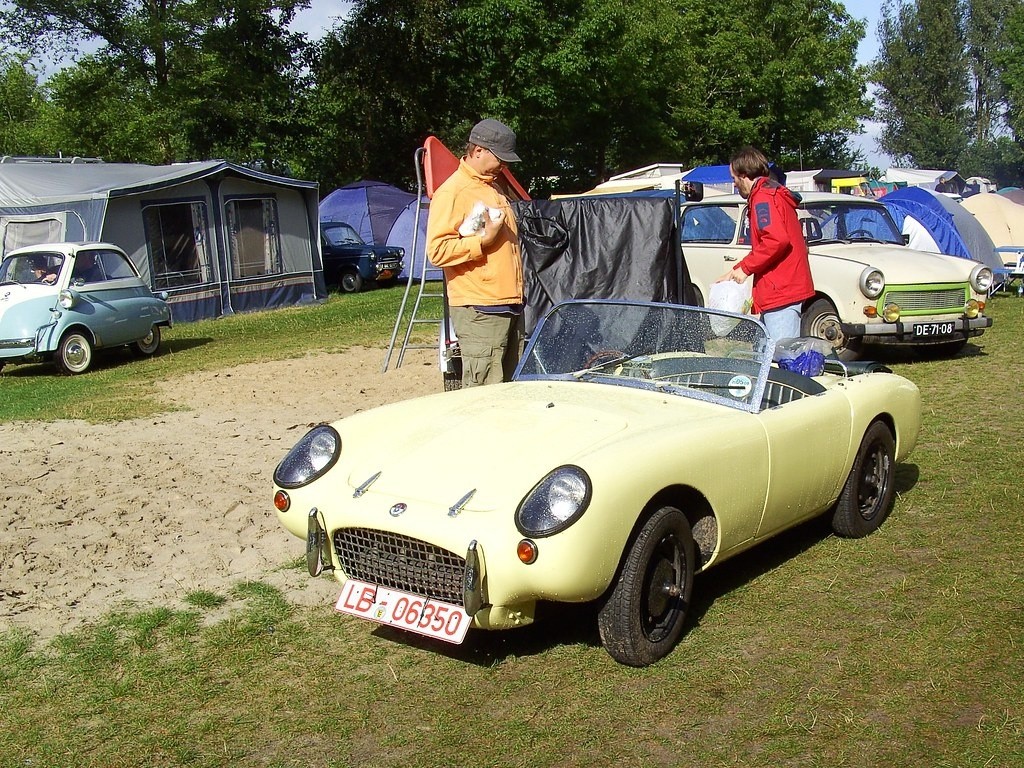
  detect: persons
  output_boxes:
[47,252,103,284]
[715,148,816,354]
[32,257,48,283]
[968,180,980,195]
[425,119,525,388]
[935,176,946,193]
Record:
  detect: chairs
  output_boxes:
[799,217,823,241]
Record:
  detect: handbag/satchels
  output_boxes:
[709,278,754,337]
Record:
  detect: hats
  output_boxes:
[469,119,522,163]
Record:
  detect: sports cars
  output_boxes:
[273,296,923,665]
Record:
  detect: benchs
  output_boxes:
[651,356,825,411]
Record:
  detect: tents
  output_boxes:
[0,156,1024,326]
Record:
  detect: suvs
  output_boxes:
[319,221,406,294]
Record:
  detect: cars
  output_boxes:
[0,239,174,379]
[672,190,995,362]
